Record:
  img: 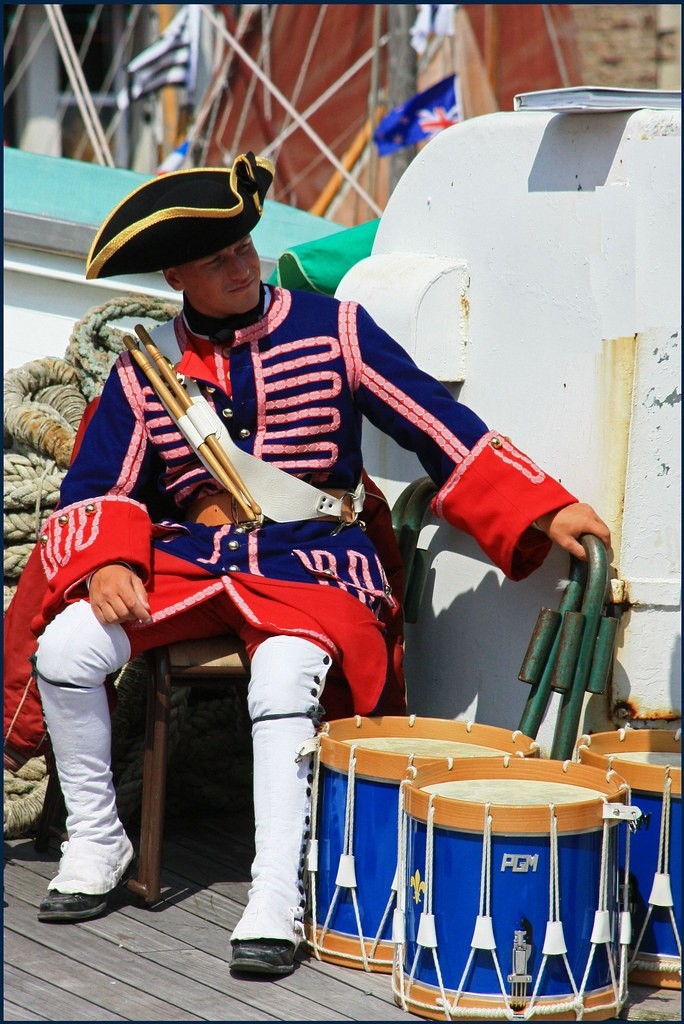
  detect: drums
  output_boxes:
[575,725,684,992]
[398,755,630,1024]
[302,714,544,973]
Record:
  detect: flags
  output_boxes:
[125,4,195,106]
[373,75,460,158]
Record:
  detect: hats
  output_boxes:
[86,147,276,280]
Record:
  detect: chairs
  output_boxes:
[33,634,347,904]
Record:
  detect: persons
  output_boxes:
[27,150,614,982]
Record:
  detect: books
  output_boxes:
[513,85,683,114]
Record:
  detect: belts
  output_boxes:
[183,489,358,526]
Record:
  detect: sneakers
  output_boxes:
[228,939,296,974]
[37,853,139,920]
